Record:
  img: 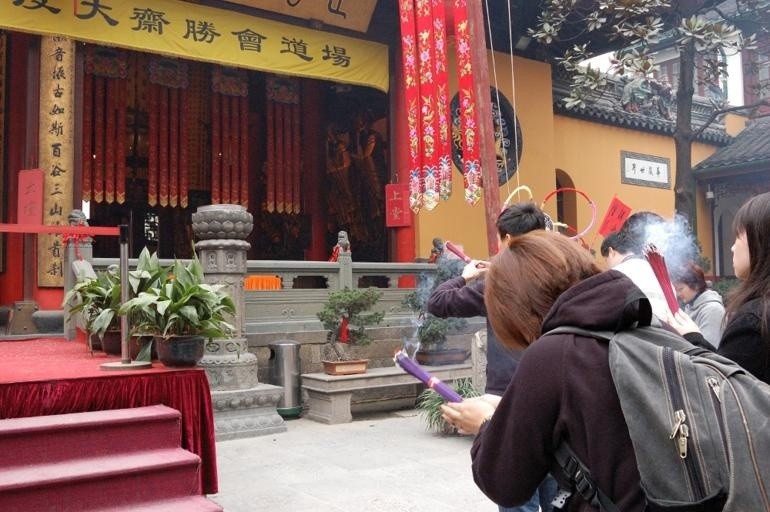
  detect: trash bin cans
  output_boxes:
[267,340,302,417]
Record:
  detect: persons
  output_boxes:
[669,259,729,351]
[598,231,673,327]
[439,231,654,510]
[429,200,547,397]
[346,108,392,263]
[669,189,768,386]
[322,124,369,253]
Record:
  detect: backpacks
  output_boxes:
[539,321,769,511]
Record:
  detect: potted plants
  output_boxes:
[415,375,483,435]
[62,238,243,368]
[401,258,472,366]
[316,286,386,376]
